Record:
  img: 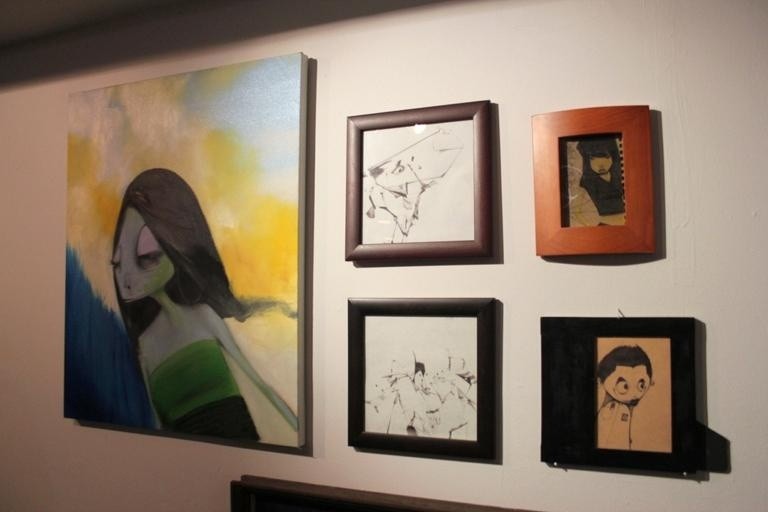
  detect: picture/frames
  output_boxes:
[345,297,502,466]
[229,475,537,512]
[346,98,493,267]
[532,105,660,258]
[538,315,708,480]
